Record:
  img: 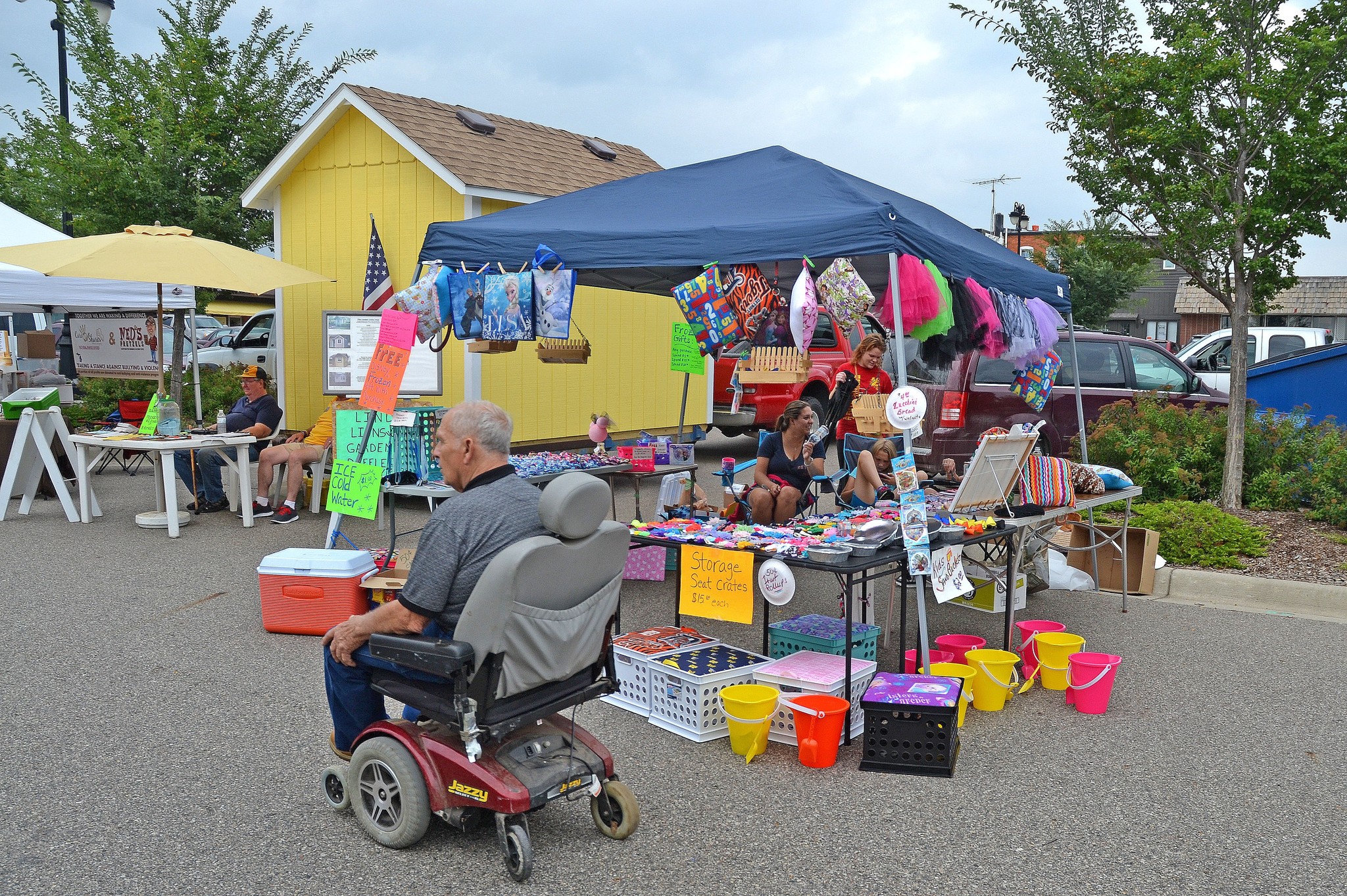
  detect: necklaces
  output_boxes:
[784,433,804,451]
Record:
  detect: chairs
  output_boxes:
[829,433,904,508]
[219,408,333,514]
[713,431,828,529]
[95,398,154,476]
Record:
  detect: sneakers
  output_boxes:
[270,504,299,524]
[896,575,932,588]
[198,491,230,512]
[186,495,207,511]
[877,486,895,500]
[235,500,274,518]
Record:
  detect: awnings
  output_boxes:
[0,201,202,428]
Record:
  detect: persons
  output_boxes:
[829,333,894,514]
[746,400,827,526]
[234,395,356,524]
[174,365,278,513]
[321,401,560,761]
[841,437,927,510]
[888,427,1009,589]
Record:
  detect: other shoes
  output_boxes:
[329,730,352,761]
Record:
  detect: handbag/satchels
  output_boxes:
[530,244,577,340]
[482,272,536,341]
[447,264,491,340]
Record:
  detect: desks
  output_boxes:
[68,428,257,538]
[932,486,1143,654]
[612,511,1018,747]
[379,454,633,528]
[0,416,77,500]
[599,464,698,521]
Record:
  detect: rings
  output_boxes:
[942,463,944,465]
[945,461,947,464]
[328,441,331,442]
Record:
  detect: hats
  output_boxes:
[235,366,267,382]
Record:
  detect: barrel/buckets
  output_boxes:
[905,649,954,675]
[918,663,977,728]
[778,692,850,768]
[934,634,987,666]
[1066,653,1122,714]
[1032,632,1086,690]
[964,649,1021,711]
[717,684,782,756]
[1015,620,1066,675]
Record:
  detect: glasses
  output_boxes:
[240,379,260,384]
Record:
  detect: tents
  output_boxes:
[324,144,1099,675]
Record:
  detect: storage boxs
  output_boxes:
[0,329,73,419]
[945,565,1027,613]
[256,548,417,635]
[617,446,655,472]
[302,476,330,506]
[636,439,672,464]
[1067,524,1160,596]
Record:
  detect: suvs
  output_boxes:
[0,308,278,381]
[880,324,1231,478]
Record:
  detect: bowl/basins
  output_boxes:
[940,524,965,540]
[839,540,882,557]
[859,518,899,548]
[927,517,943,541]
[891,521,902,545]
[805,545,853,563]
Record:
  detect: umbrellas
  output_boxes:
[0,220,338,399]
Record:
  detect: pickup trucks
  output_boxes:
[712,306,891,438]
[1130,326,1336,395]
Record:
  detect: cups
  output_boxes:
[721,458,736,473]
[840,521,851,536]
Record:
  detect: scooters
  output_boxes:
[316,469,642,882]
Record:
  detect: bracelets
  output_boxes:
[804,457,814,467]
[300,431,306,438]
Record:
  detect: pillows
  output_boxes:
[1019,455,1134,508]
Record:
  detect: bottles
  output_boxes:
[1033,447,1042,456]
[156,398,180,436]
[806,425,829,444]
[217,410,227,434]
[746,512,753,525]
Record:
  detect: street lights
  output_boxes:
[1009,201,1031,255]
